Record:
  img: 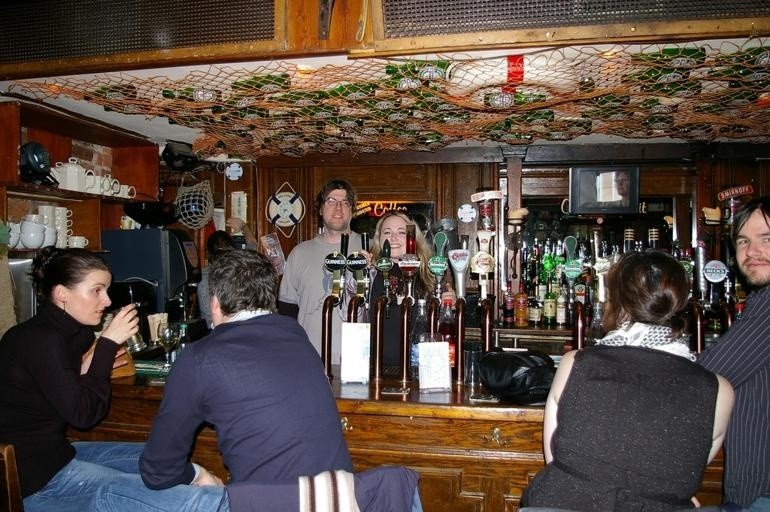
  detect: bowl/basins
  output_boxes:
[7,222,57,249]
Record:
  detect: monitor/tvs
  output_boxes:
[569,165,641,215]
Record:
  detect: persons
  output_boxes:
[519,250,736,512]
[0,245,147,512]
[197,217,258,329]
[369,212,436,294]
[134,250,354,512]
[277,180,375,364]
[695,196,770,512]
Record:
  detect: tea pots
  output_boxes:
[114,185,136,198]
[86,170,110,194]
[50,157,95,192]
[102,174,120,196]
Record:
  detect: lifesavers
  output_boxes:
[445,56,577,105]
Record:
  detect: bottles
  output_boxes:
[542,280,556,325]
[530,244,540,279]
[515,279,528,326]
[591,303,603,326]
[178,323,191,351]
[554,240,566,281]
[703,283,722,347]
[532,237,540,256]
[529,280,543,326]
[557,282,568,326]
[583,286,593,326]
[520,239,529,266]
[541,242,553,280]
[436,299,457,380]
[409,299,431,380]
[573,274,585,304]
[503,281,515,328]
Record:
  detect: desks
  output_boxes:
[73,358,726,512]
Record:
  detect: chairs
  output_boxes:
[0,444,25,512]
[227,468,413,512]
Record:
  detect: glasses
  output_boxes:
[325,198,350,207]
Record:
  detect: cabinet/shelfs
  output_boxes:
[1,93,160,332]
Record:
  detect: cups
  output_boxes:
[113,306,147,352]
[158,324,182,367]
[26,206,55,229]
[121,220,131,229]
[419,333,445,344]
[68,236,88,248]
[55,208,73,248]
[463,349,482,386]
